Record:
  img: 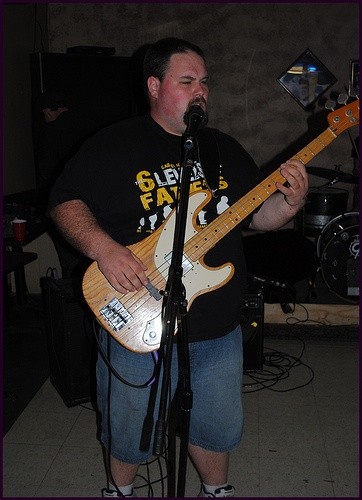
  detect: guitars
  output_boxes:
[81,84,362,355]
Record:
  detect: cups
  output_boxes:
[11,220,28,241]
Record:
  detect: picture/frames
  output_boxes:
[275,48,337,111]
[348,59,360,99]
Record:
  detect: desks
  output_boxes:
[5,251,38,306]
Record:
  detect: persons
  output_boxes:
[43,38,311,498]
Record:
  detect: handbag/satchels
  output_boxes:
[41,267,78,337]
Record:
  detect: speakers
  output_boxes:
[39,273,99,407]
[240,288,264,372]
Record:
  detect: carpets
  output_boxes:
[3,294,55,435]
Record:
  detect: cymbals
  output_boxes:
[304,166,355,184]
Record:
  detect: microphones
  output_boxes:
[184,105,204,149]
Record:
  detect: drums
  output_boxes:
[296,186,349,237]
[316,211,360,304]
